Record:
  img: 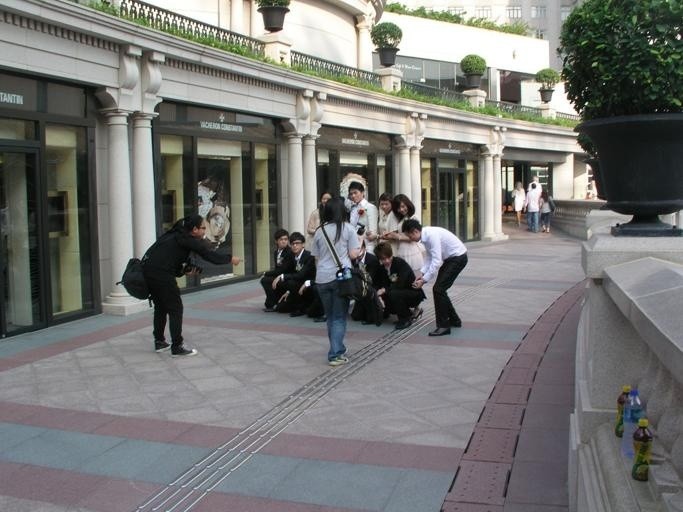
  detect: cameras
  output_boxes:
[182,256,204,275]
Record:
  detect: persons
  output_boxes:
[511,182,525,229]
[526,175,542,225]
[260,181,468,367]
[538,192,554,233]
[525,184,539,233]
[140,215,246,358]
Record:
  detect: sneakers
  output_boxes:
[328,356,349,365]
[155,341,172,353]
[345,345,352,358]
[170,347,198,357]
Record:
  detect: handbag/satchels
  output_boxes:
[116,257,154,306]
[336,268,366,301]
[548,197,555,211]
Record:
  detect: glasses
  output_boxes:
[199,226,206,230]
[291,241,300,245]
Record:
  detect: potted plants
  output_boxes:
[369,22,401,69]
[557,0,683,239]
[460,55,486,87]
[535,67,559,102]
[256,0,292,33]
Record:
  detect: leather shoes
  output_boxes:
[428,328,450,336]
[265,307,423,330]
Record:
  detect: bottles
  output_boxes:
[337,273,343,281]
[615,385,630,436]
[622,391,642,457]
[629,420,653,479]
[204,164,229,252]
[342,265,351,279]
